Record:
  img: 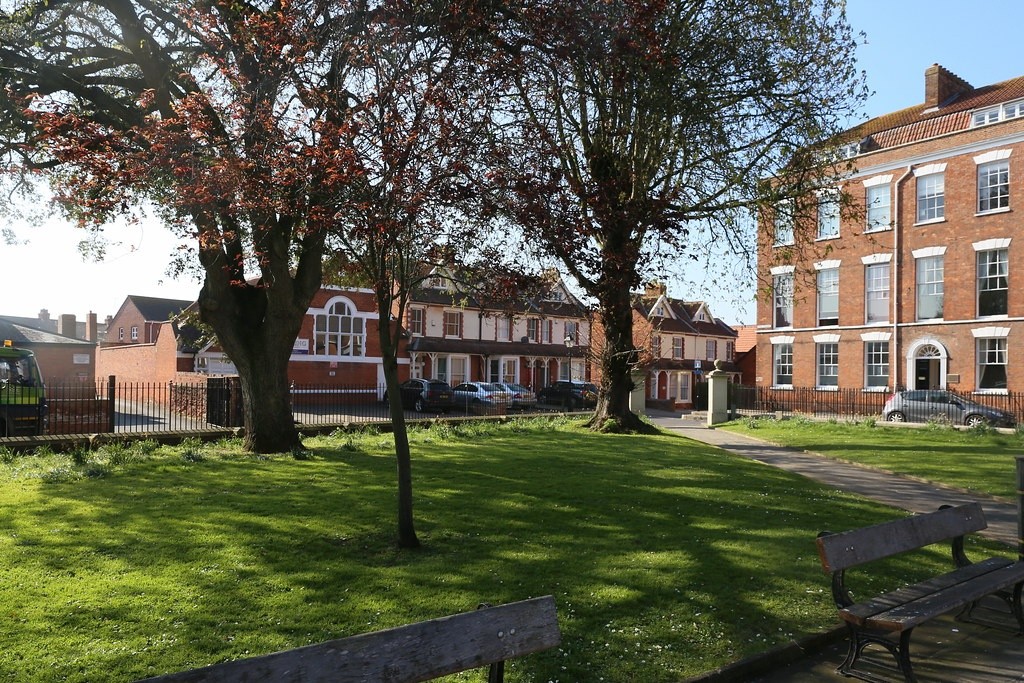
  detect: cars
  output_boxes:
[450,382,513,416]
[492,382,538,412]
[881,389,1018,428]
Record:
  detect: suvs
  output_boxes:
[383,377,454,414]
[538,378,598,410]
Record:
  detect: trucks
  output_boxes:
[0,340,48,456]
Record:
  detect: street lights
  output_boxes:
[563,331,575,412]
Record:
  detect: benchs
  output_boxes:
[130,596,564,683]
[817,498,1023,683]
